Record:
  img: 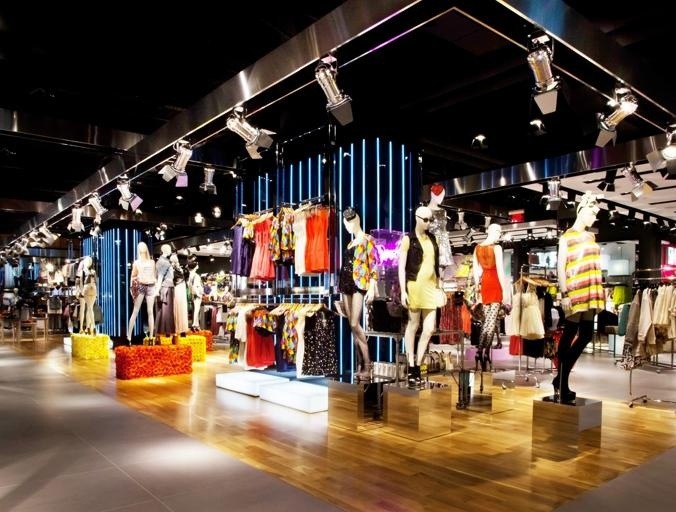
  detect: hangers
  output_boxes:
[267,304,341,319]
[636,280,675,288]
[514,271,561,286]
[230,196,329,230]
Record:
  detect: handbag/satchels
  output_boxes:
[368,297,403,333]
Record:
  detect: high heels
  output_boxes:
[414,364,421,382]
[407,366,417,387]
[356,360,374,382]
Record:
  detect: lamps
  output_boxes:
[645,122,676,172]
[67,203,85,234]
[4,220,60,257]
[540,180,567,210]
[314,54,354,125]
[591,86,639,150]
[85,192,109,215]
[606,201,676,232]
[116,173,144,212]
[526,34,557,116]
[621,162,658,201]
[157,139,194,188]
[226,106,279,160]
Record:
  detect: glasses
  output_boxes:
[416,215,434,223]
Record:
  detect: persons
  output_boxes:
[185,261,204,331]
[75,255,93,334]
[423,183,456,345]
[119,241,159,344]
[337,208,380,383]
[153,244,178,346]
[395,205,440,386]
[471,222,508,372]
[78,257,98,335]
[550,196,606,407]
[167,254,190,337]
